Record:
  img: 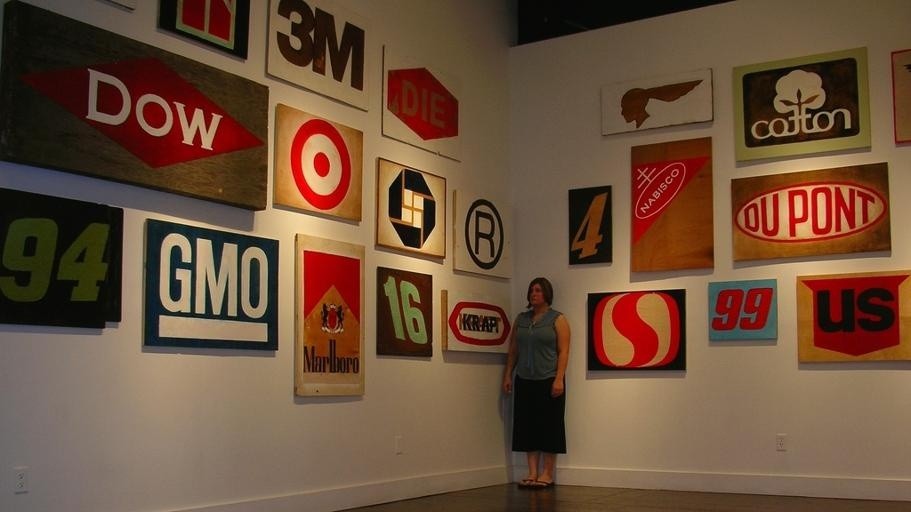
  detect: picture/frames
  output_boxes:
[729,47,872,162]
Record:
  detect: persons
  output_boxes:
[503,277,572,490]
[520,485,556,511]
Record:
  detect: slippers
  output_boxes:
[518,476,556,489]
